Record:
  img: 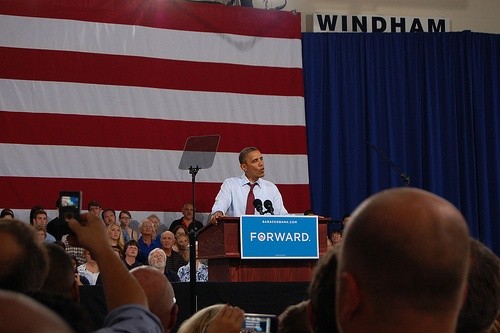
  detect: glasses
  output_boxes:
[142,224,151,229]
[121,217,130,220]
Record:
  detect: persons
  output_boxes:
[129,266,308,333]
[0,201,209,285]
[209,147,323,227]
[0,213,165,333]
[309,188,500,333]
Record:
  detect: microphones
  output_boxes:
[253,198,264,215]
[264,200,274,215]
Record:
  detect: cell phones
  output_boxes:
[58,190,82,234]
[239,312,280,333]
